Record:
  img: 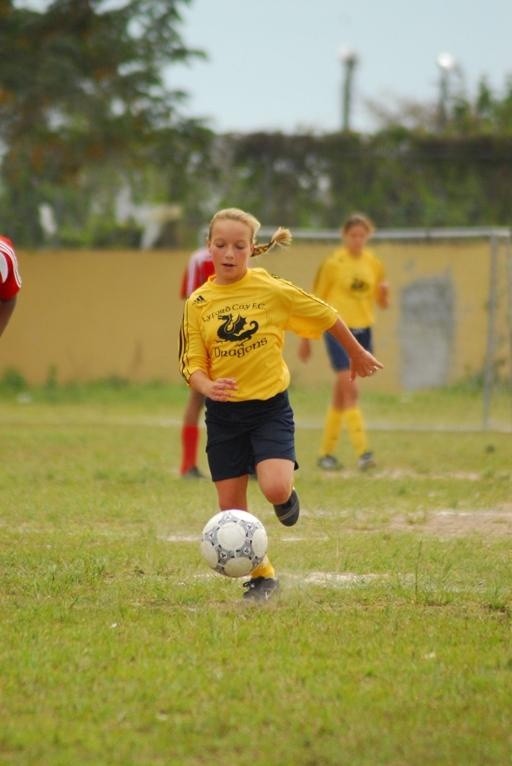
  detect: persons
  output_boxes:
[179,209,385,609]
[176,247,258,484]
[296,212,392,474]
[0,235,21,337]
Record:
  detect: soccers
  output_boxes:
[200,509,268,577]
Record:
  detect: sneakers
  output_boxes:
[235,577,280,602]
[182,465,209,480]
[319,451,375,470]
[273,487,302,525]
